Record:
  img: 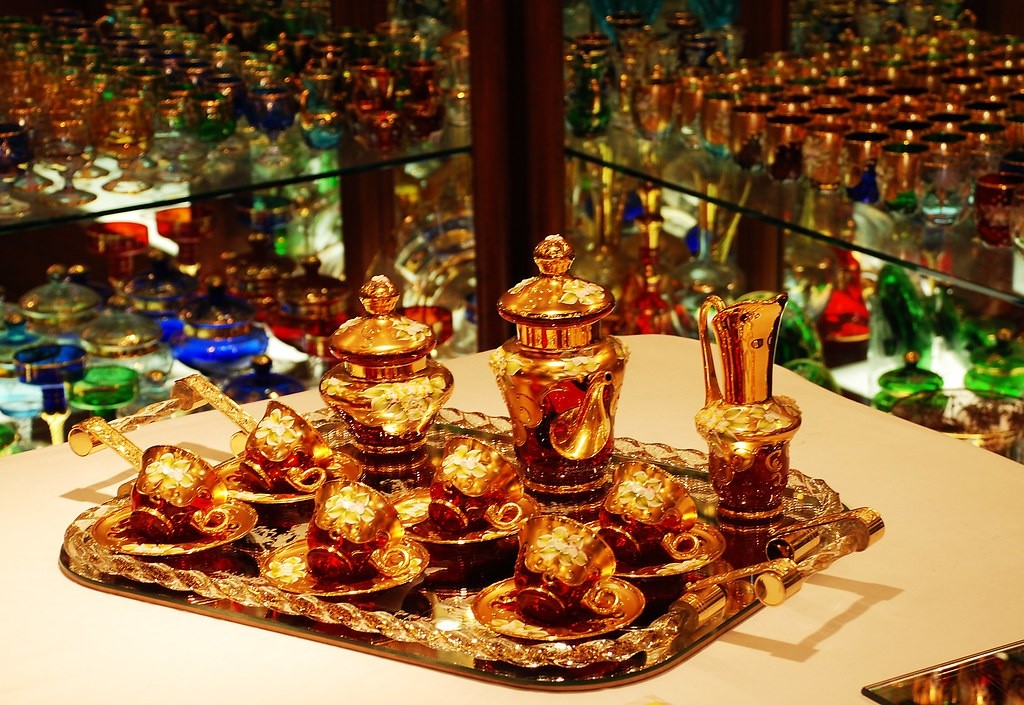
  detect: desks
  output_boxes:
[2,331,1023,704]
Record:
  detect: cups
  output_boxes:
[428,435,522,532]
[130,447,234,540]
[157,0,1023,252]
[602,464,700,560]
[512,515,617,621]
[241,401,334,492]
[307,481,410,581]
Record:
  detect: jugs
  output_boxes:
[695,294,802,521]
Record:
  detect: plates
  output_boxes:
[262,537,429,596]
[215,452,363,504]
[473,577,644,639]
[583,519,725,577]
[93,501,256,555]
[392,491,542,545]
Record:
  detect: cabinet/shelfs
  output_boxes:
[2,2,1020,428]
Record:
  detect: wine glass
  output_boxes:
[1,0,297,281]
